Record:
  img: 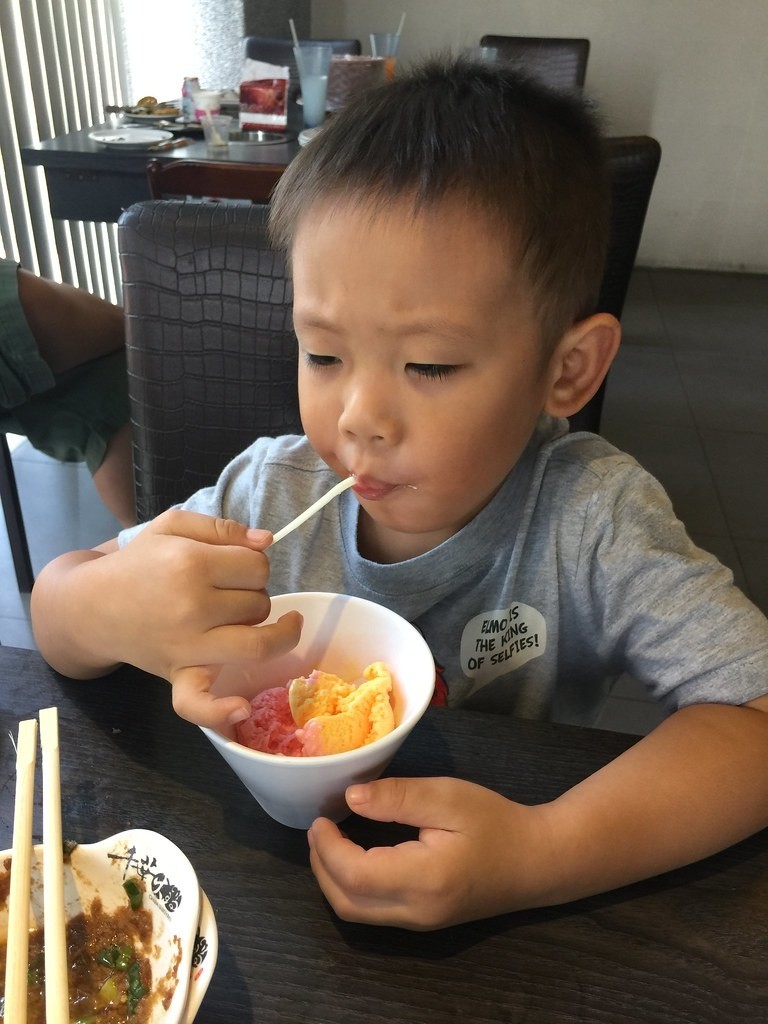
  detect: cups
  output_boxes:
[191,92,223,124]
[293,45,333,130]
[370,32,399,81]
[199,115,233,153]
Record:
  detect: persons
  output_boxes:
[30,46,768,930]
[0,256,135,526]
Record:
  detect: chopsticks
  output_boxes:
[1,705,72,1024]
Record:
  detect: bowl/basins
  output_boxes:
[175,888,218,1023]
[198,591,436,831]
[1,824,200,1024]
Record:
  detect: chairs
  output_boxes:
[477,34,591,94]
[243,36,361,114]
[115,130,663,529]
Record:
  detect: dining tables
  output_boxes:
[2,645,767,1024]
[23,116,324,222]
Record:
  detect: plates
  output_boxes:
[87,128,173,152]
[122,109,186,124]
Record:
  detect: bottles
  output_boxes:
[182,77,200,122]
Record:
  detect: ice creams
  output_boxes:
[236,663,399,759]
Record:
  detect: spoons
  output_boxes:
[260,476,357,553]
